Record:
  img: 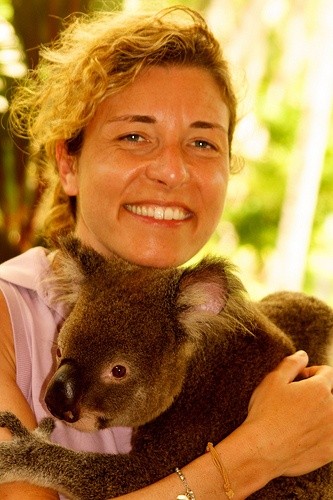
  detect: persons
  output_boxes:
[0,6,332,500]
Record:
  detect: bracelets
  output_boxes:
[175,468,193,499]
[207,443,233,498]
